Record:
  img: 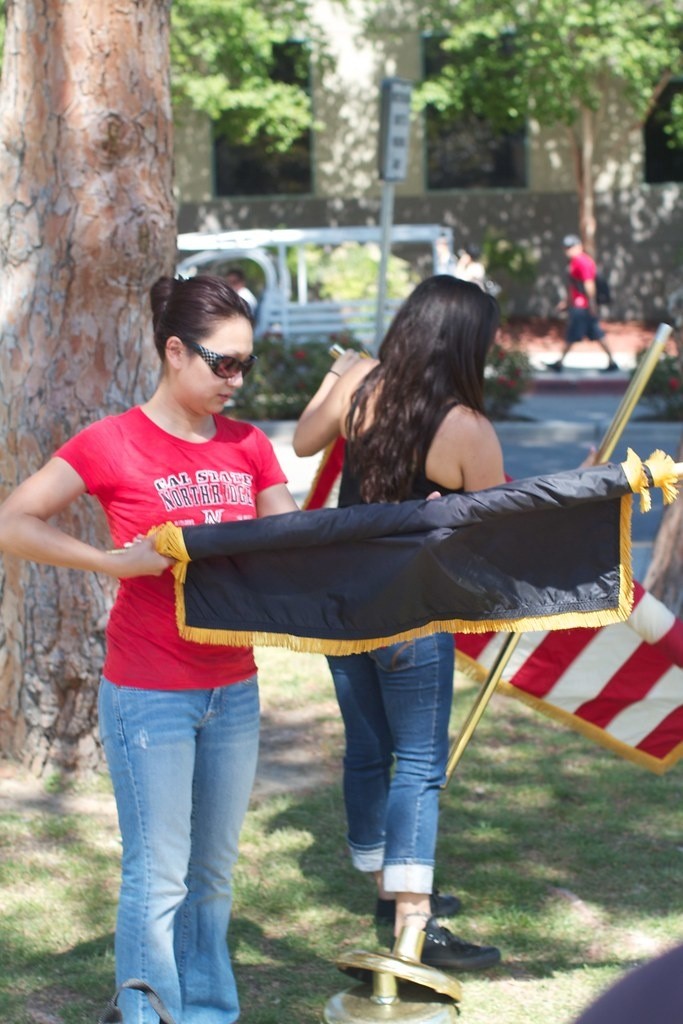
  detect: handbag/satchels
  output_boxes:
[565,263,610,303]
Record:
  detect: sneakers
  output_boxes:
[392,915,500,970]
[375,887,462,926]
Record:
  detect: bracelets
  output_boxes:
[328,369,341,378]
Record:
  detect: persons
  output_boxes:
[1,275,299,1024]
[224,270,258,315]
[292,275,509,969]
[543,235,620,373]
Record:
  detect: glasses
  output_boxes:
[182,338,256,378]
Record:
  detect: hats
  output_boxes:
[561,233,579,250]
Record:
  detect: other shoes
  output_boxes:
[543,362,564,372]
[597,363,619,373]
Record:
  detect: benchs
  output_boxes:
[268,294,406,337]
